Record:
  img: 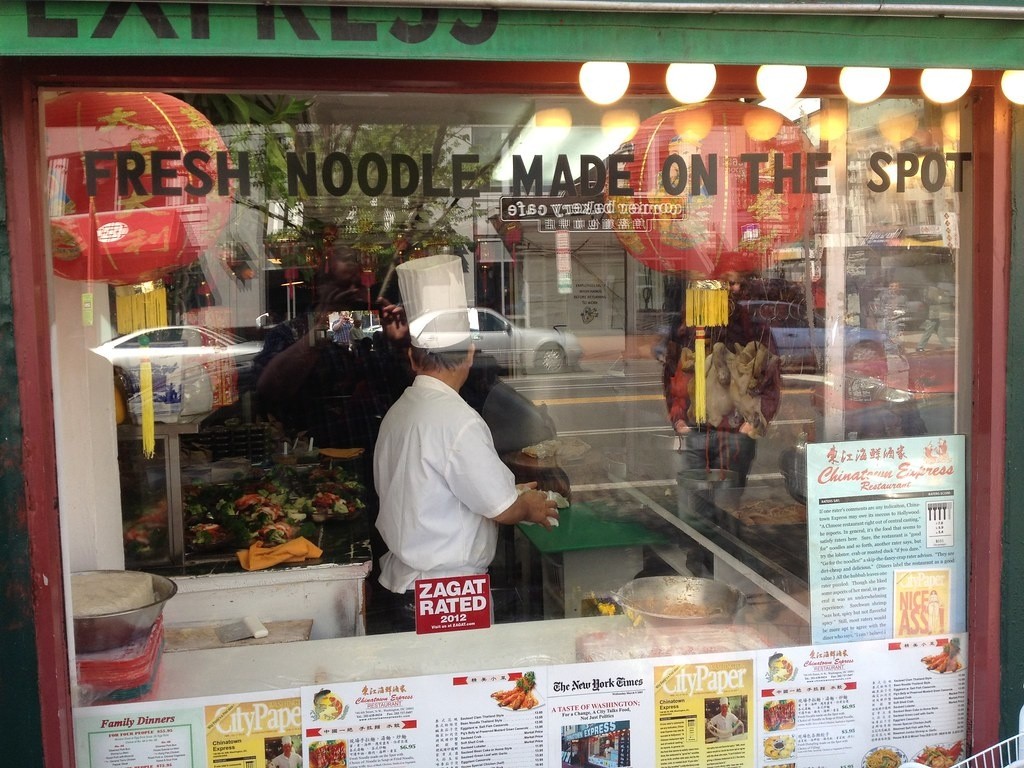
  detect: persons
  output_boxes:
[373,296,559,632]
[332,311,365,342]
[710,698,742,739]
[260,281,418,486]
[604,740,613,758]
[916,286,951,352]
[876,281,907,350]
[662,269,780,520]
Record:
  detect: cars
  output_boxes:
[808,347,954,415]
[87,325,269,418]
[779,394,953,509]
[654,300,888,376]
[406,306,583,377]
[362,324,382,333]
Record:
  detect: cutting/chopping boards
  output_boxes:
[501,446,602,475]
[163,617,314,653]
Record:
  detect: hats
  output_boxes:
[396,254,469,348]
[352,312,361,320]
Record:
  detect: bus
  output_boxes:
[767,232,958,354]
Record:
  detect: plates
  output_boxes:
[860,744,908,768]
[899,762,931,768]
[494,685,546,712]
[909,742,955,763]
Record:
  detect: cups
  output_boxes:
[512,655,565,668]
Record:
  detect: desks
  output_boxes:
[516,503,669,553]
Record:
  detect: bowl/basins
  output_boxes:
[273,452,300,465]
[705,736,720,743]
[296,447,322,464]
[69,569,178,654]
[614,576,747,629]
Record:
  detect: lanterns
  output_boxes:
[606,100,816,423]
[41,91,234,459]
